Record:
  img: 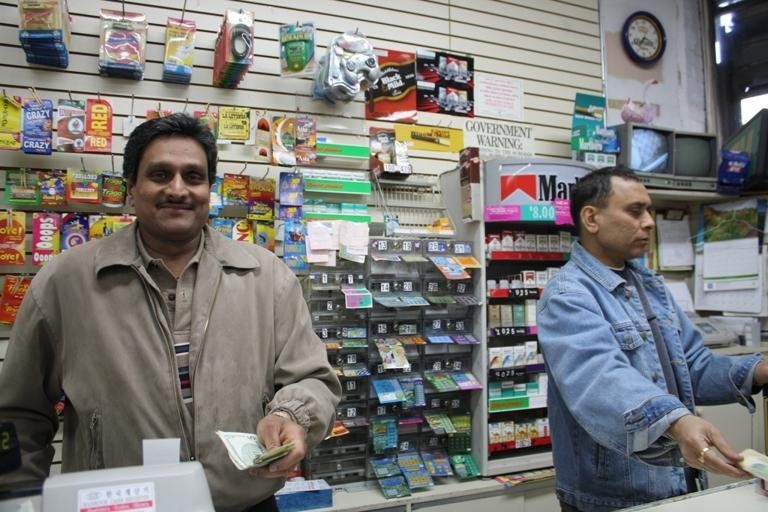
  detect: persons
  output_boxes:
[535,165,767,510]
[0,110,346,512]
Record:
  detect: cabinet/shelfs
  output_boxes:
[694,388,766,490]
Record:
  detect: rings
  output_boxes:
[698,446,712,464]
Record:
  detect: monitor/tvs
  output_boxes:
[607,122,676,189]
[674,131,720,192]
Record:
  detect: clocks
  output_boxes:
[620,9,666,67]
